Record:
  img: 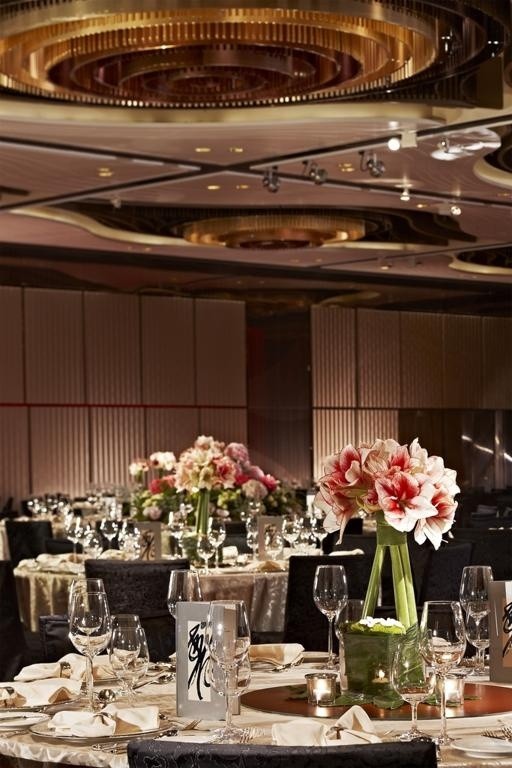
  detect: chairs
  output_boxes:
[1,486,512,766]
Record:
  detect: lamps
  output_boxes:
[259,152,384,194]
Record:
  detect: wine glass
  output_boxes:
[310,561,367,691]
[162,566,207,662]
[25,483,332,575]
[417,598,467,744]
[458,562,498,679]
[389,630,436,743]
[202,597,261,744]
[66,577,151,716]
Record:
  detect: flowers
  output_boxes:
[309,434,470,634]
[130,426,277,558]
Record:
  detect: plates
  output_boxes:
[298,649,338,664]
[0,711,53,733]
[448,733,512,760]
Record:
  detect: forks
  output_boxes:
[157,711,201,731]
